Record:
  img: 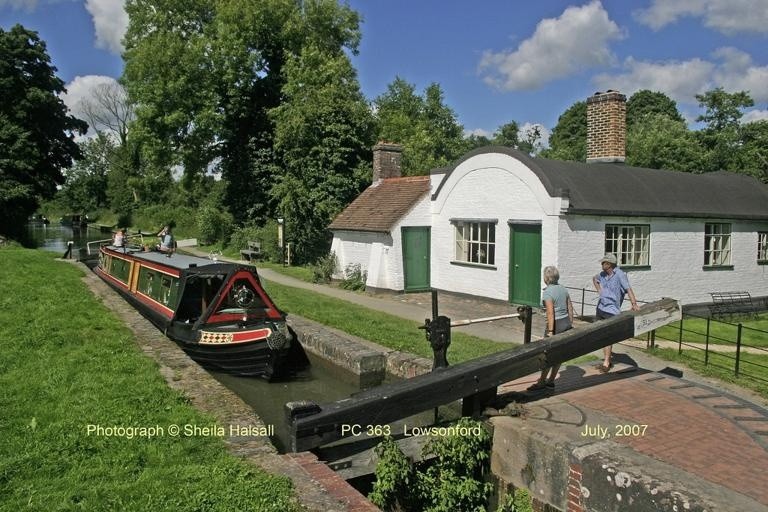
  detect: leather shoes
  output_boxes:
[599,363,612,374]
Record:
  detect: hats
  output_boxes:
[599,254,617,263]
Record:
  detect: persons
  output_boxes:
[155,226,175,254]
[112,227,127,248]
[525,265,573,391]
[591,252,640,370]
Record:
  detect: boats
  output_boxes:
[29,214,50,225]
[92,241,294,382]
[61,212,87,229]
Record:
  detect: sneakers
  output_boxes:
[527,383,546,392]
[545,383,555,390]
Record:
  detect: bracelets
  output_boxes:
[630,302,636,306]
[547,330,554,333]
